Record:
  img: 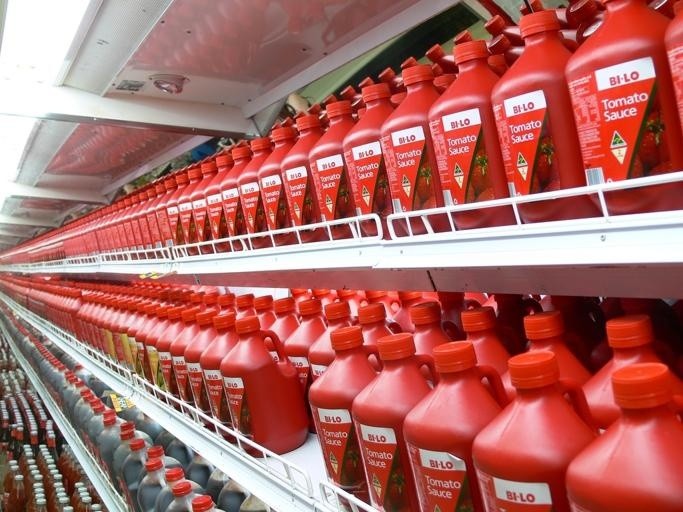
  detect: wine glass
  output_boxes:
[0,0,682,267]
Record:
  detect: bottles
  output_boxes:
[0,332,104,512]
[0,275,252,511]
[252,273,683,512]
